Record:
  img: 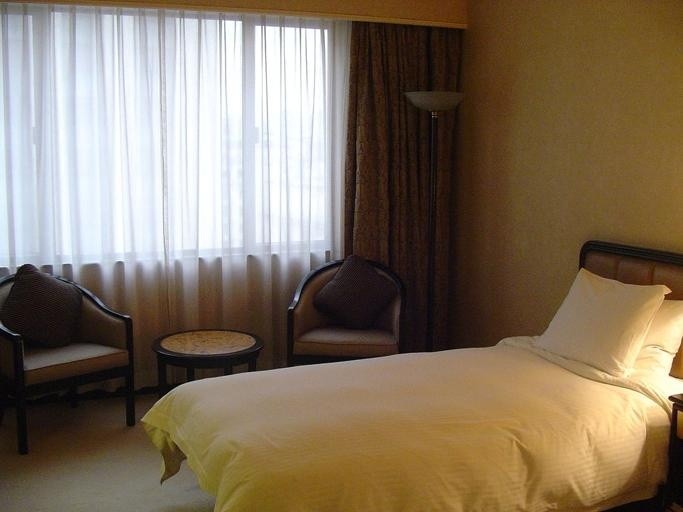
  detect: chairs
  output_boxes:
[0,269,135,455]
[287,258,406,366]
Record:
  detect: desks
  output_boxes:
[152,329,265,395]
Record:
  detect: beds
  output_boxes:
[140,241,683,512]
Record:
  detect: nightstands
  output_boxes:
[667,394,683,512]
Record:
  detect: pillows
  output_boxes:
[0,265,83,351]
[537,268,683,388]
[313,254,396,330]
